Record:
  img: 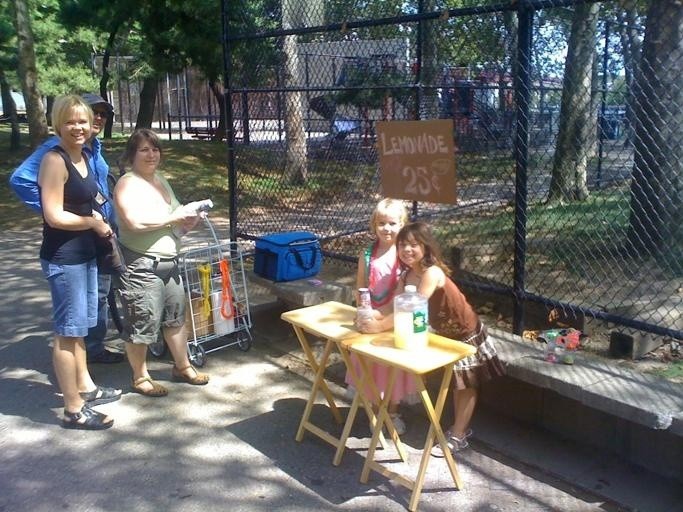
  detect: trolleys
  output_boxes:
[148,210,252,368]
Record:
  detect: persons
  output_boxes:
[114,128,209,398]
[355,223,506,458]
[10,94,116,365]
[356,197,408,438]
[39,94,122,432]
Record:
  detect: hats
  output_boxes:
[79,93,114,113]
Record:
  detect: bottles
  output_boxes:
[356,287,372,331]
[392,283,430,352]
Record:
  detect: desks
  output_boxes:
[340,327,477,512]
[281,301,389,467]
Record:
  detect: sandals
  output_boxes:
[129,374,169,398]
[78,385,122,409]
[387,412,406,437]
[435,425,473,444]
[59,403,114,431]
[368,414,387,435]
[430,432,469,457]
[170,362,210,386]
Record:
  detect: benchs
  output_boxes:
[482,324,683,434]
[186,127,235,140]
[245,269,347,307]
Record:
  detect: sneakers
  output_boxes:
[87,349,126,364]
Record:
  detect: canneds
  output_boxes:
[356,287,371,307]
